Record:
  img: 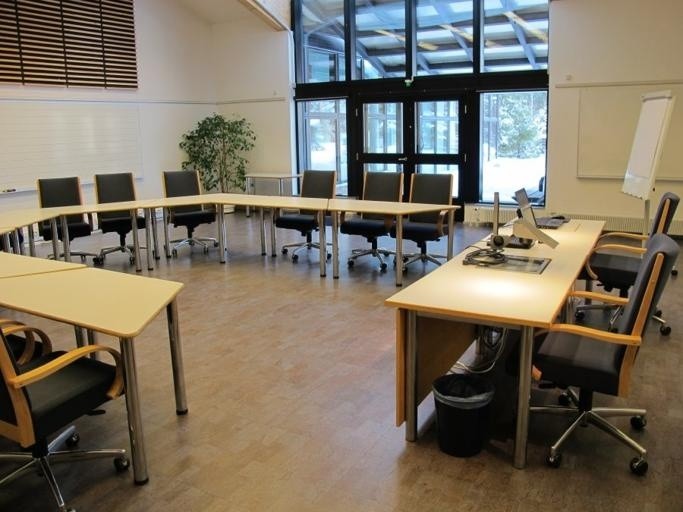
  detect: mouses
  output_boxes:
[551,215,564,220]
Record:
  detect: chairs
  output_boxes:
[275,170,454,274]
[0,317,131,511]
[34,169,218,268]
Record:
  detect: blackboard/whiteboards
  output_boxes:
[576,80,683,181]
[621,88,677,201]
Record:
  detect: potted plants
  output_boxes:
[178,111,256,214]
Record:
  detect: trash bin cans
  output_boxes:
[432,374,496,457]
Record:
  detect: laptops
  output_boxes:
[514,187,564,229]
[489,204,538,249]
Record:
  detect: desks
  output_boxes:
[384,216,606,470]
[244,172,302,218]
[0,266,188,486]
